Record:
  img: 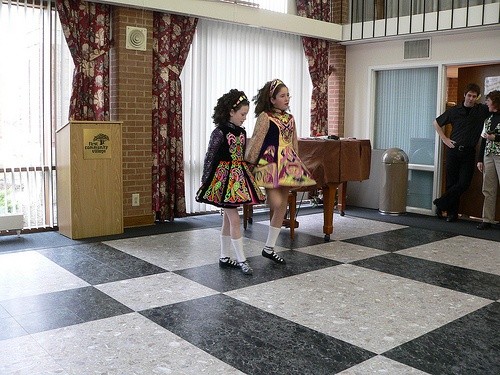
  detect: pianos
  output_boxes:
[243,137,371,241]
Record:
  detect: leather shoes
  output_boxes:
[446,212,456,222]
[433,199,442,218]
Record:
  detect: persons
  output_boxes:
[432,84,493,222]
[476,89,500,229]
[195,88,266,275]
[244,79,317,264]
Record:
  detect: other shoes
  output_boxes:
[262,246,286,264]
[239,260,254,275]
[477,220,491,229]
[219,257,238,267]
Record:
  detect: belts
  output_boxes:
[454,144,471,152]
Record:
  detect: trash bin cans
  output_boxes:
[378,147,410,216]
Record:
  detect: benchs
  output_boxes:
[0,215,24,238]
